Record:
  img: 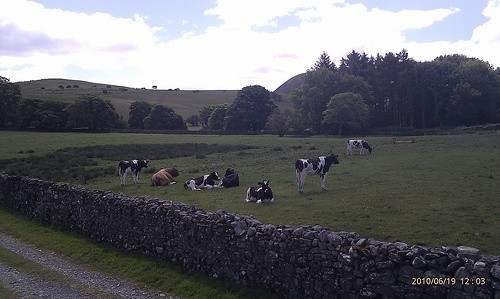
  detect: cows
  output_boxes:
[220,168,239,187]
[296,154,339,193]
[347,140,372,156]
[244,180,273,203]
[117,159,149,186]
[184,172,221,191]
[151,167,181,188]
